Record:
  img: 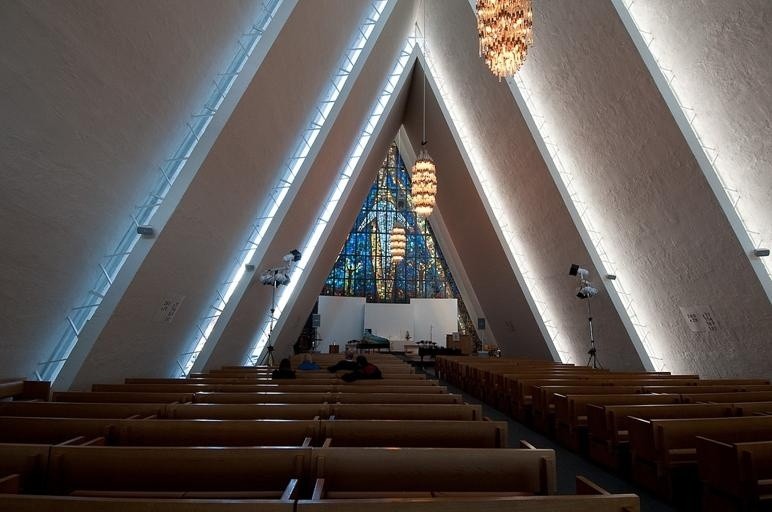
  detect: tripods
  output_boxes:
[586,296,603,369]
[259,282,276,366]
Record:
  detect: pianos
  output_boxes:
[356,331,390,353]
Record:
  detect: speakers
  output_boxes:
[312,314,320,327]
[477,317,485,329]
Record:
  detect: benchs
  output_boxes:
[0,349,643,512]
[432,351,771,509]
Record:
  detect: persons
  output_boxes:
[271,359,296,379]
[344,355,383,382]
[328,350,357,373]
[298,354,321,370]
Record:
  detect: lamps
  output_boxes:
[409,1,440,222]
[472,1,535,84]
[566,263,603,370]
[258,247,307,366]
[388,142,408,266]
